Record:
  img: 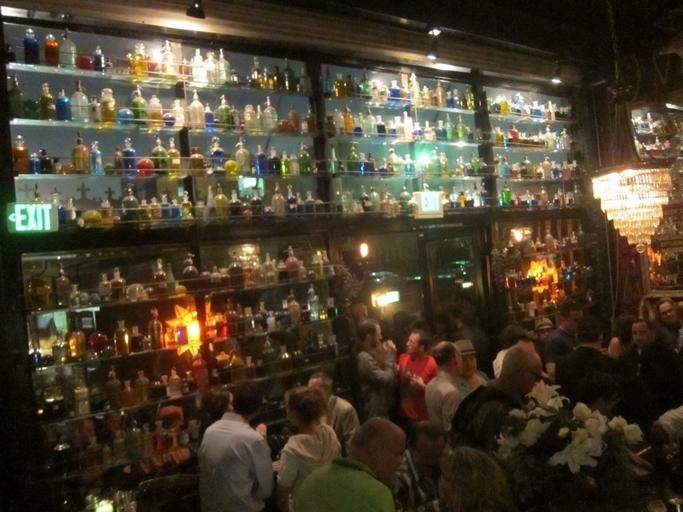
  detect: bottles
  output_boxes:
[506,230,595,311]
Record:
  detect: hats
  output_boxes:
[534,317,554,331]
[455,339,479,356]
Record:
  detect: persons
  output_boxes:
[306,373,360,457]
[198,384,274,509]
[296,417,406,509]
[272,388,342,497]
[199,387,234,441]
[347,290,681,510]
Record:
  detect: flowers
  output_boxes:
[493,380,644,473]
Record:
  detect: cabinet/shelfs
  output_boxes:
[623,90,683,300]
[0,5,604,426]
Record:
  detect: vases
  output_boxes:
[581,453,614,488]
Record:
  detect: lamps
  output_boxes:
[590,165,672,255]
